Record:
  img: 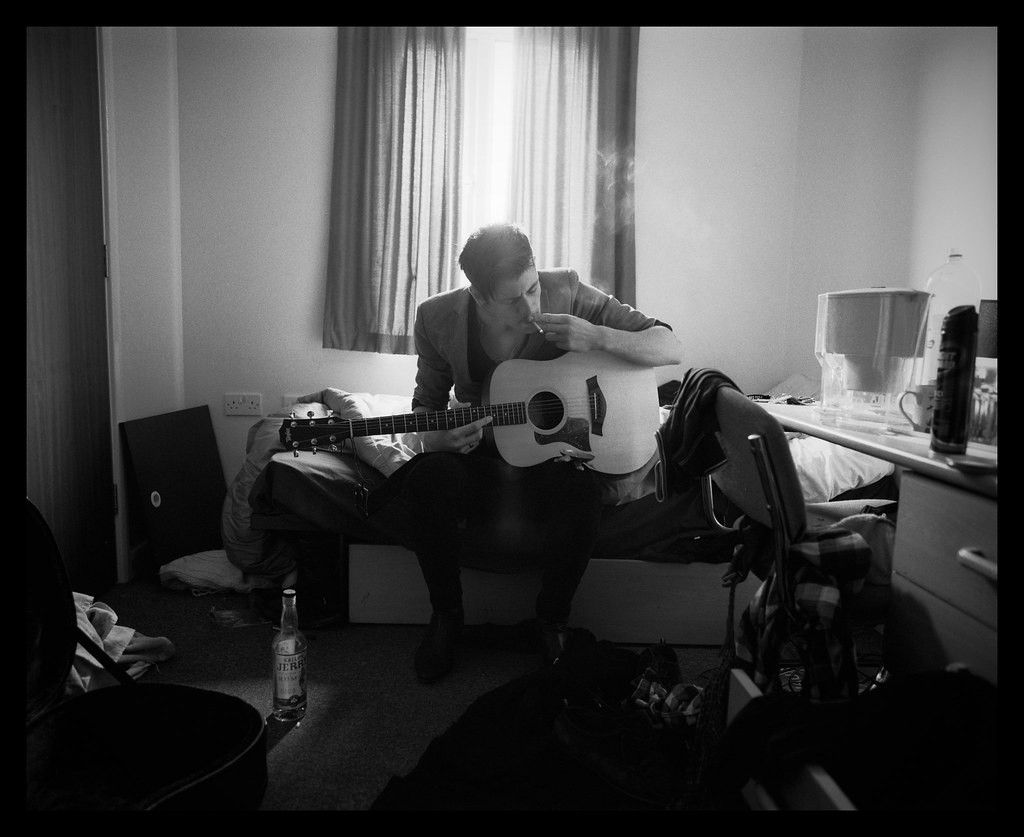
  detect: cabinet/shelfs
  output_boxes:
[757,403,999,733]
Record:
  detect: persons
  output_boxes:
[403,222,683,681]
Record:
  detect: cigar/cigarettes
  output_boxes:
[532,320,544,333]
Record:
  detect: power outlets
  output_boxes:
[224,392,263,416]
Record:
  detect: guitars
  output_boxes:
[278,348,663,476]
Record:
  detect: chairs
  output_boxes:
[652,367,894,698]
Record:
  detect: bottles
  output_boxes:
[271,589,309,722]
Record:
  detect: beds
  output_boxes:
[219,372,901,649]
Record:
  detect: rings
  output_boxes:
[469,444,474,448]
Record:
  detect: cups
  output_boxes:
[929,304,981,454]
[886,393,913,436]
[897,384,933,433]
